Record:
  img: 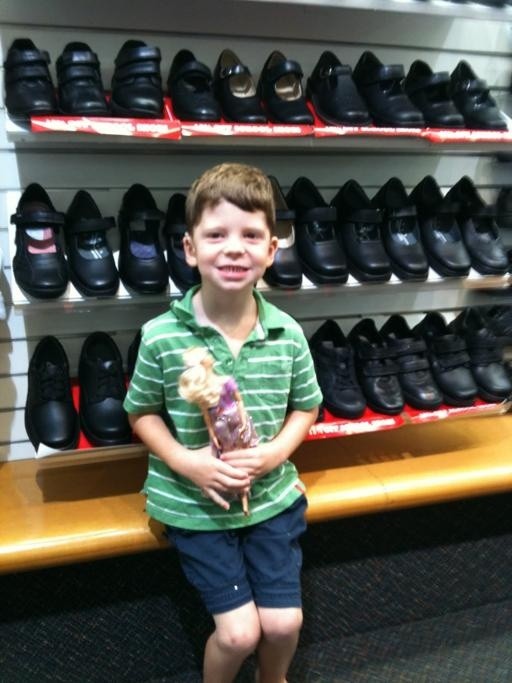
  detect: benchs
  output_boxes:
[0,412,511,580]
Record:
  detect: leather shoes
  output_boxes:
[25,332,79,450]
[309,305,512,419]
[79,332,132,446]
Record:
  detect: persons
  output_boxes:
[116,159,326,682]
[176,345,262,516]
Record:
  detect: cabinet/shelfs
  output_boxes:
[0,0,512,471]
